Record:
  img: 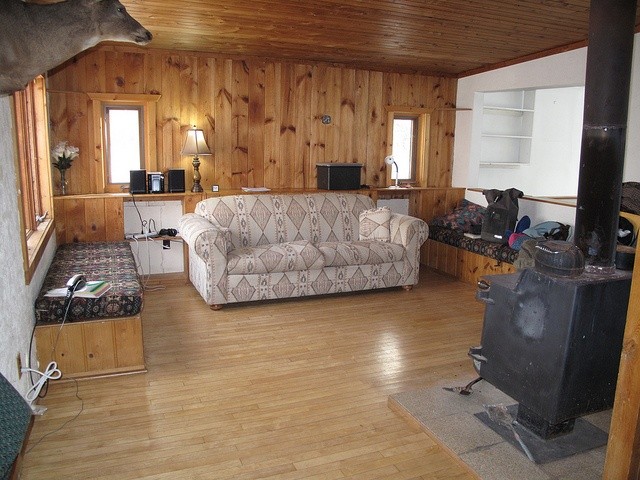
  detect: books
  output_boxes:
[242,186,271,192]
[45,280,105,295]
[463,232,482,239]
[40,281,112,299]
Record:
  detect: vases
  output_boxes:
[56,167,70,196]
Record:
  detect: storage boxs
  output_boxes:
[316,163,364,190]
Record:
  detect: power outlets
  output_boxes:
[17,354,23,379]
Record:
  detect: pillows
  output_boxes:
[215,225,232,252]
[431,200,487,235]
[358,206,391,241]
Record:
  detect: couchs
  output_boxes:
[178,193,430,311]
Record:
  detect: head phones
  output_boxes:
[159,228,177,236]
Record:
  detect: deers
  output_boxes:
[0,0,154,95]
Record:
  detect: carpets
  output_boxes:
[391,380,613,479]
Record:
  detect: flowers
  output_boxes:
[53,141,81,186]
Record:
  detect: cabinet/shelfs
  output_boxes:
[473,88,538,167]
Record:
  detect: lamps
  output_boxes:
[385,155,404,190]
[180,129,213,193]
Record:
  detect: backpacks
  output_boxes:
[481,188,522,244]
[508,221,572,250]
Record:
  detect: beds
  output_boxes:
[35,241,147,380]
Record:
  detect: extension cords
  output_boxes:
[134,231,159,239]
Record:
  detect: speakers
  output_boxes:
[147,172,165,194]
[168,167,185,192]
[129,169,146,194]
